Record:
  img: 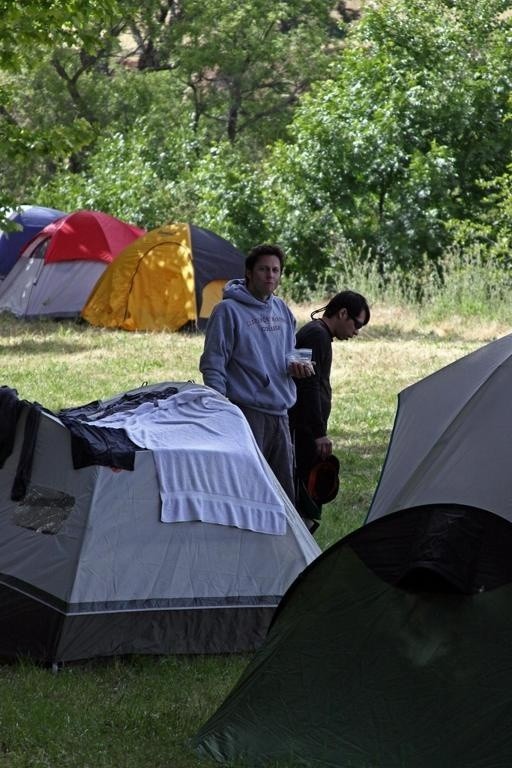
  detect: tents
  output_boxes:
[362,333,512,528]
[194,503,512,768]
[79,220,244,333]
[0,214,53,279]
[0,378,322,663]
[0,210,144,319]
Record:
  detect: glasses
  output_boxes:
[350,315,362,330]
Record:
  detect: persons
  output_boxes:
[286,290,369,532]
[197,243,318,504]
